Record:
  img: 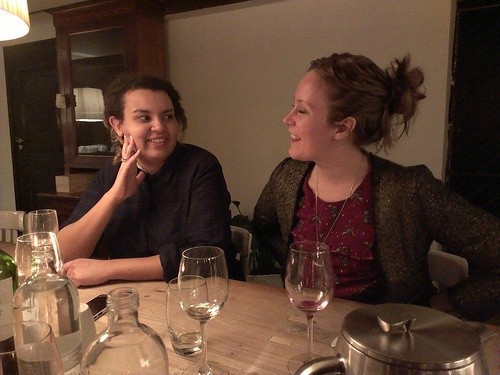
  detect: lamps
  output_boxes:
[0,0,31,42]
[72,86,105,122]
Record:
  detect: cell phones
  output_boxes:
[79,293,110,323]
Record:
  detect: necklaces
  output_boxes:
[316,151,364,261]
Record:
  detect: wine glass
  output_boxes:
[285,240,337,375]
[177,246,230,375]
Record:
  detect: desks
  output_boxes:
[0,242,500,375]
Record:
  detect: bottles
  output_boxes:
[79,286,170,375]
[10,244,83,375]
[0,248,20,375]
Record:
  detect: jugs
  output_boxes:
[292,302,497,375]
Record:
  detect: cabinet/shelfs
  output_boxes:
[54,0,168,176]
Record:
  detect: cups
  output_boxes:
[0,320,65,375]
[27,209,59,236]
[166,274,209,357]
[14,232,63,287]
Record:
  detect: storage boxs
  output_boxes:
[57,171,93,194]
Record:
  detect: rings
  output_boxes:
[120,159,126,161]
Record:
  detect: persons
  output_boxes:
[250,52,500,323]
[56,72,246,288]
